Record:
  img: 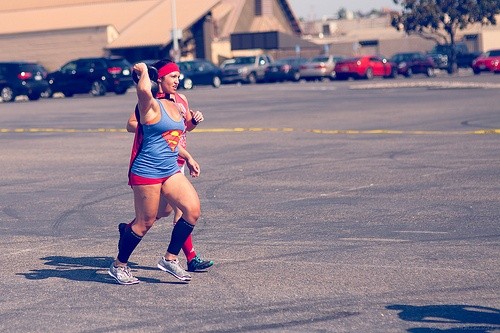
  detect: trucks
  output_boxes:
[429,42,484,72]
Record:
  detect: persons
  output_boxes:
[108,62,200,284]
[118,59,213,271]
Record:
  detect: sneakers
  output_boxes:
[156,255,192,281]
[187,253,214,272]
[108,262,139,285]
[118,222,130,249]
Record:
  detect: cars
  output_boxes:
[218,53,438,85]
[176,61,224,90]
[472,49,500,74]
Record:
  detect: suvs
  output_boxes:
[0,61,52,102]
[46,55,133,96]
